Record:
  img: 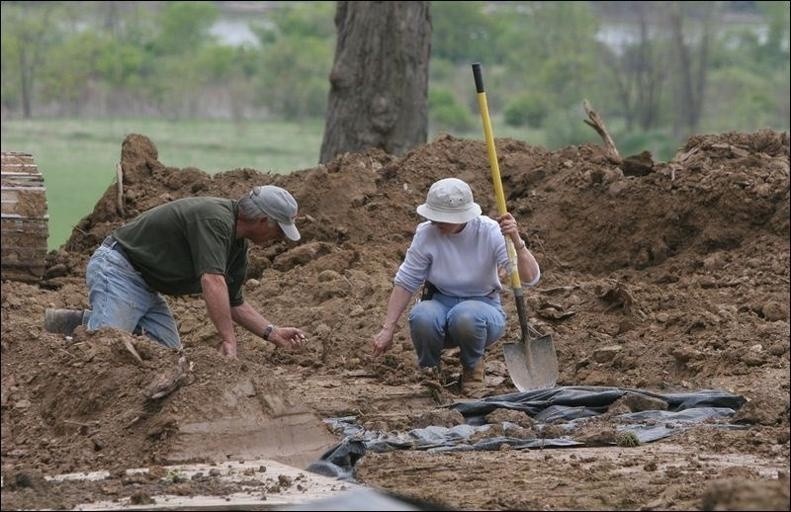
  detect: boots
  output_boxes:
[45,308,84,335]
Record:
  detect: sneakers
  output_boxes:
[461,360,484,398]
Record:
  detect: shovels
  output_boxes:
[471,61,560,391]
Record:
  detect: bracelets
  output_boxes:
[516,241,525,250]
[264,323,275,340]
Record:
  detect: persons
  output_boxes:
[45,185,307,358]
[374,177,540,392]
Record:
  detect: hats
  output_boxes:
[249,185,301,242]
[416,178,482,224]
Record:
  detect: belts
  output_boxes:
[105,238,129,260]
